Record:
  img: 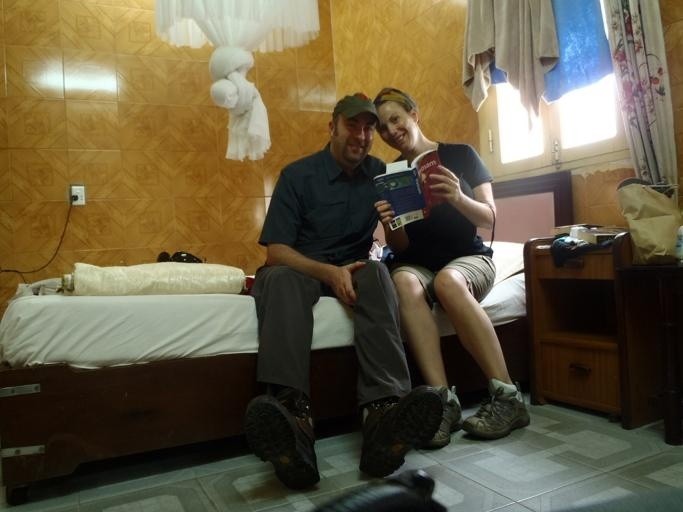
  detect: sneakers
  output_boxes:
[458,381,529,440]
[428,385,463,449]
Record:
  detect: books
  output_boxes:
[372,148,449,231]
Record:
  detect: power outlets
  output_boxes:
[71,185,86,206]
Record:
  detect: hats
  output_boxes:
[331,93,380,131]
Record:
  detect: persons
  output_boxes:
[243,92,442,489]
[369,87,528,449]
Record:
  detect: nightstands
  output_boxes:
[522,231,676,431]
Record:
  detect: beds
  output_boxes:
[0,168,574,509]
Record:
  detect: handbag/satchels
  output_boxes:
[616,183,682,266]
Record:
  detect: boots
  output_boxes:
[243,383,319,491]
[359,386,445,478]
